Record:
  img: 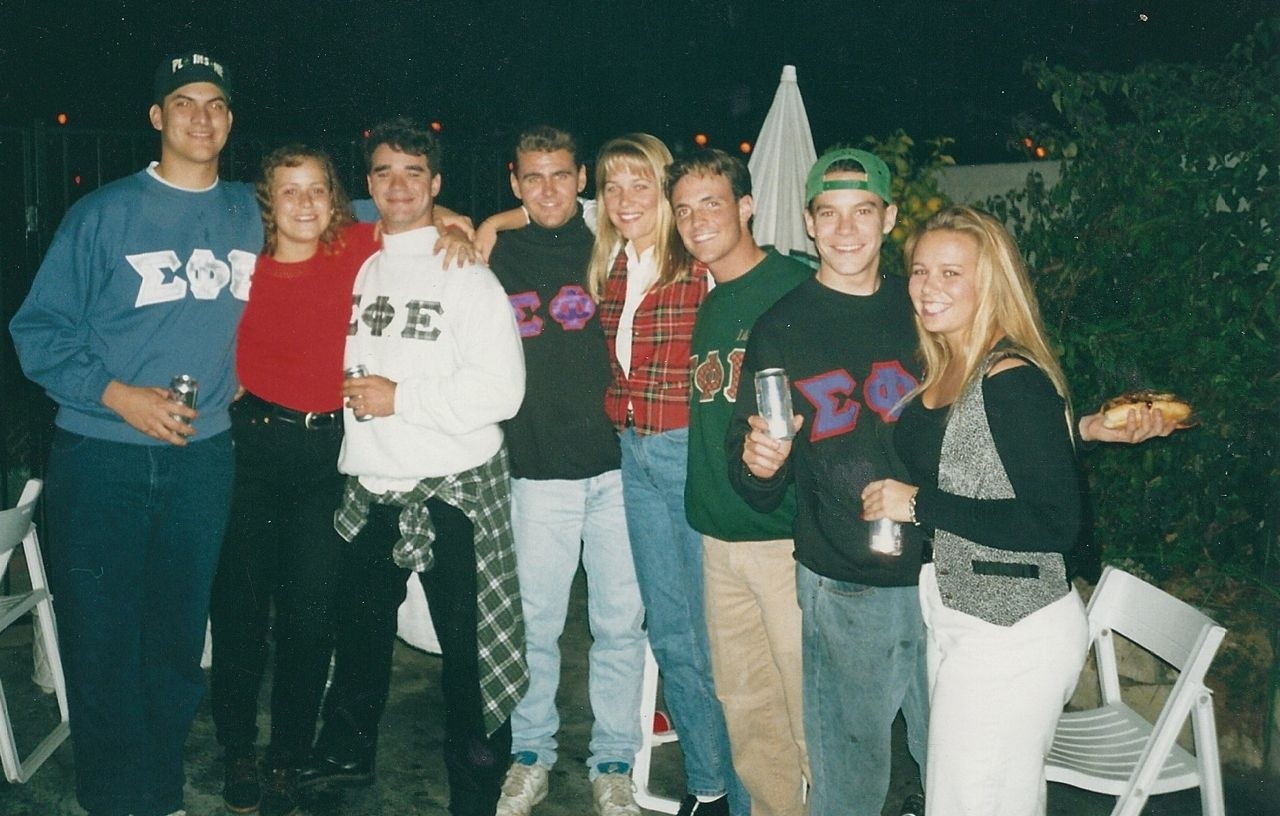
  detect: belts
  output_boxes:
[240,391,340,429]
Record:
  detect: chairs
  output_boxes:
[1044,562,1228,816]
[0,479,71,783]
[632,645,681,815]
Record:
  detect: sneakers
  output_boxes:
[494,759,548,816]
[591,773,642,816]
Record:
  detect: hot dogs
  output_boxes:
[1099,392,1199,429]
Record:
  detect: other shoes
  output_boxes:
[299,761,376,783]
[261,766,302,816]
[222,745,261,812]
[676,793,729,816]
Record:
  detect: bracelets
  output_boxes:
[484,219,497,230]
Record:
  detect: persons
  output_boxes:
[475,133,744,814]
[266,117,524,814]
[484,129,658,816]
[217,132,474,814]
[728,148,1179,814]
[8,44,269,816]
[661,147,815,815]
[860,203,1102,814]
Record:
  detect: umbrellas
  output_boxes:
[745,65,818,259]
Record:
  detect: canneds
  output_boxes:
[868,518,904,556]
[344,364,378,421]
[167,374,198,438]
[754,367,796,441]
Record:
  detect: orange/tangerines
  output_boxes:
[888,195,943,242]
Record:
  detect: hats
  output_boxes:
[151,52,231,107]
[805,149,893,208]
